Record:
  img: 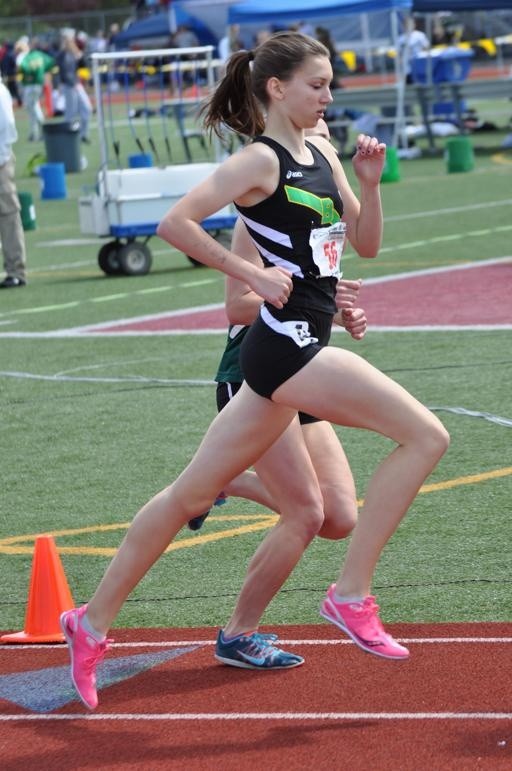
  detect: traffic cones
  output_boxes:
[2,535,75,644]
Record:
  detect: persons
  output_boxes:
[395,18,432,85]
[219,23,240,60]
[14,36,56,141]
[48,27,93,143]
[60,33,451,710]
[179,119,367,669]
[1,81,27,284]
[173,26,200,59]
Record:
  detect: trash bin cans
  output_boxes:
[411,49,476,126]
[380,145,398,182]
[43,122,81,172]
[446,136,473,173]
[129,153,153,166]
[38,164,67,201]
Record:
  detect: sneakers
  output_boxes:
[60,603,110,710]
[187,492,227,531]
[0,276,25,288]
[214,628,305,670]
[319,583,410,660]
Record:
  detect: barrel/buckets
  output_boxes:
[441,136,475,173]
[127,152,152,168]
[37,161,68,199]
[379,147,402,183]
